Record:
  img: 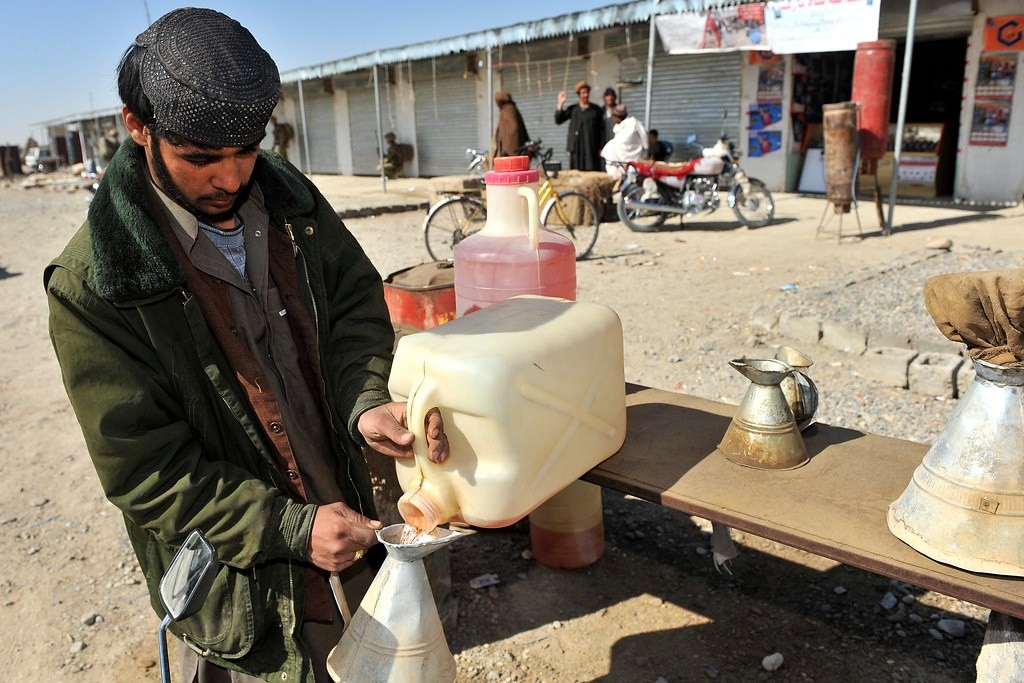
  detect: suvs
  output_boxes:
[24,146,56,171]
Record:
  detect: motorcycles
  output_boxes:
[605,111,774,232]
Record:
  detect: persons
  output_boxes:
[377,132,403,179]
[599,105,649,181]
[271,117,289,158]
[601,88,618,173]
[491,92,533,171]
[647,130,666,161]
[44,8,449,683]
[555,81,604,171]
[107,130,120,151]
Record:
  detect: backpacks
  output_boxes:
[395,144,415,161]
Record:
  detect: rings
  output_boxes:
[352,550,363,561]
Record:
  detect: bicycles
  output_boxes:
[425,139,600,262]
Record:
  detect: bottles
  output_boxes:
[453,155,576,320]
[527,482,605,571]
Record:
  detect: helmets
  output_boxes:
[386,132,395,138]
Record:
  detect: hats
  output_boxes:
[134,6,282,144]
[613,104,628,116]
[574,80,589,93]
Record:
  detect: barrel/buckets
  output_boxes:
[388,293,626,532]
[381,259,456,329]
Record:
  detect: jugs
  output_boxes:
[719,345,818,471]
[327,524,475,683]
